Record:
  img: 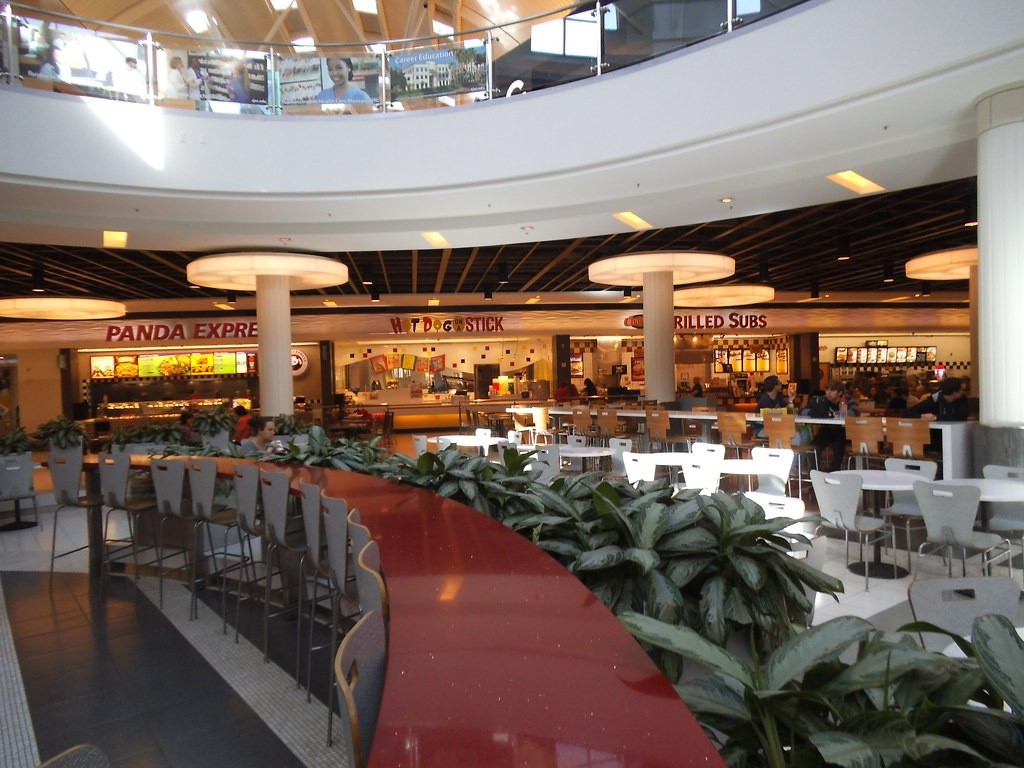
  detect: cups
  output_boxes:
[275,440,284,453]
[833,411,842,418]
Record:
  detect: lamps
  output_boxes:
[811,291,819,298]
[227,297,236,303]
[371,293,380,302]
[624,289,631,298]
[484,292,493,300]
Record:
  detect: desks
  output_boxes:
[540,444,615,467]
[637,450,701,490]
[40,452,724,768]
[825,470,924,580]
[713,458,780,487]
[926,477,1024,588]
[427,434,501,454]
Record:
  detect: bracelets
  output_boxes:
[935,416,937,420]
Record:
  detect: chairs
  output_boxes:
[0,411,1024,768]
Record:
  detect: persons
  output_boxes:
[316,58,370,104]
[36,45,70,81]
[168,56,210,99]
[848,384,932,409]
[28,28,44,53]
[231,406,253,446]
[227,63,248,103]
[808,380,860,471]
[53,33,67,50]
[905,377,971,422]
[752,375,807,477]
[554,380,569,403]
[584,378,596,396]
[179,412,194,441]
[689,377,703,398]
[240,416,281,455]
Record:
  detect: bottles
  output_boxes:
[839,397,847,419]
